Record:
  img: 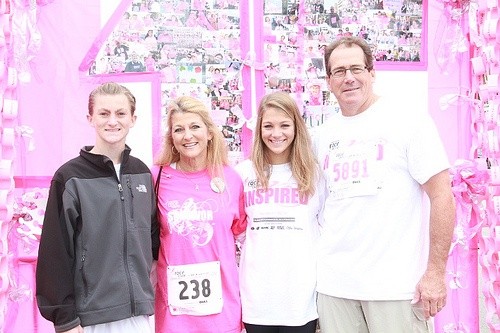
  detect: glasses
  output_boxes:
[329,66,368,75]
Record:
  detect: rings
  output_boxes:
[437,305,443,309]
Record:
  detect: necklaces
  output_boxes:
[176,161,209,191]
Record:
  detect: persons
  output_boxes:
[233,92,326,333]
[36,82,161,333]
[308,37,457,333]
[88,0,421,148]
[150,96,248,333]
